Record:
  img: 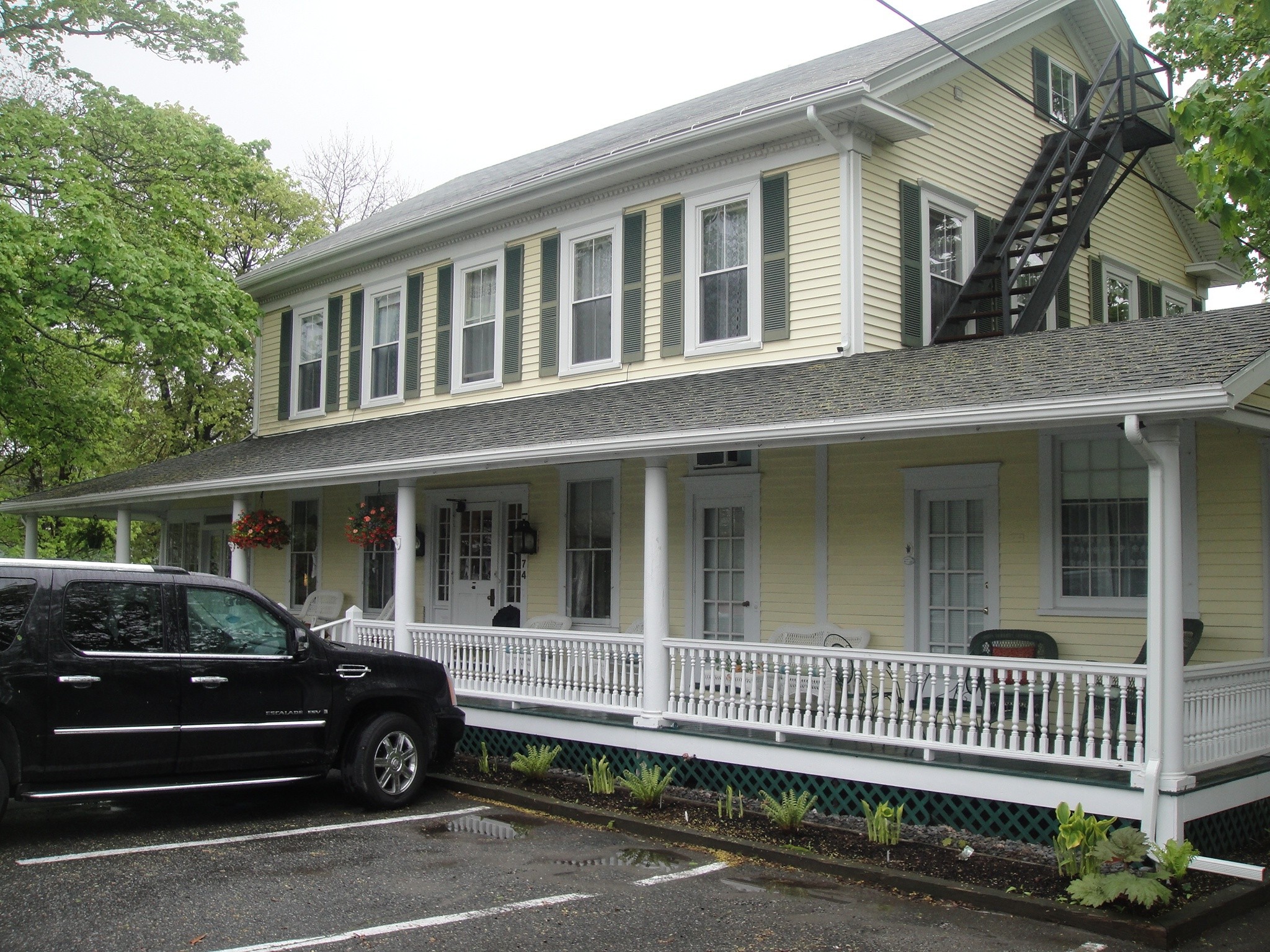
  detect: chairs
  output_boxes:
[1079,619,1204,752]
[376,594,395,620]
[506,614,644,685]
[118,602,163,652]
[824,634,902,750]
[899,639,992,761]
[292,589,344,630]
[970,629,1059,743]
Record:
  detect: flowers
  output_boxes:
[344,501,397,548]
[227,508,293,552]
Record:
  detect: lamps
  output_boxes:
[512,513,537,555]
[416,523,425,557]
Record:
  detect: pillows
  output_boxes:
[992,646,1037,685]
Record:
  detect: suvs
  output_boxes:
[0,558,466,824]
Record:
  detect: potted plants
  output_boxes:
[64,515,116,558]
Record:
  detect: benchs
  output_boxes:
[705,623,870,705]
